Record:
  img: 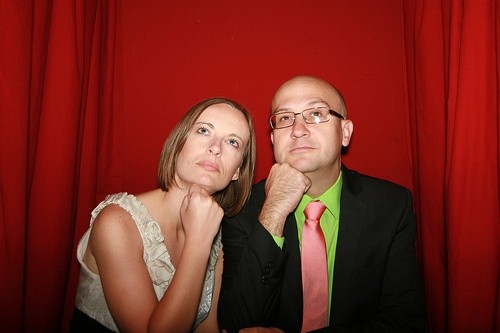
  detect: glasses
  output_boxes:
[268,106,344,130]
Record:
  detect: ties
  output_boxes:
[300,200,332,333]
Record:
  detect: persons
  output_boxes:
[64,97,257,333]
[215,75,430,333]
